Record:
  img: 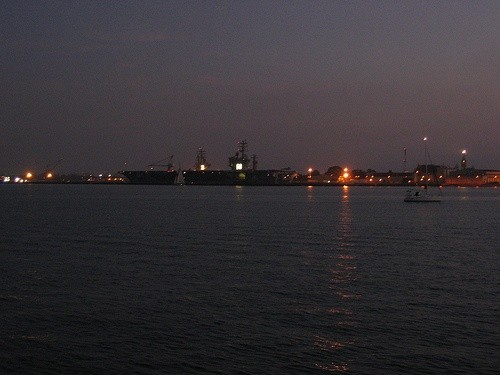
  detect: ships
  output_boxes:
[118,140,297,186]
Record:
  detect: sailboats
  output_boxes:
[403,140,469,202]
